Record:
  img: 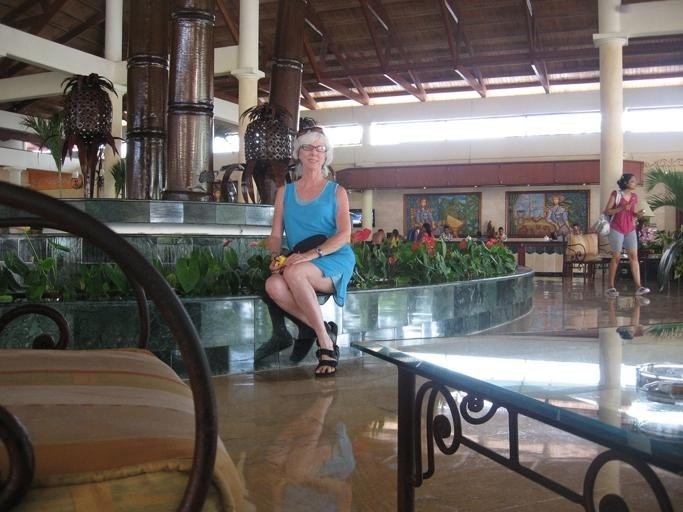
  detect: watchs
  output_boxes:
[315,246,323,258]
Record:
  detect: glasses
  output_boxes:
[302,143,327,152]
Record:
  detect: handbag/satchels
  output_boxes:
[293,235,329,253]
[591,215,610,237]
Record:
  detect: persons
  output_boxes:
[264,129,356,378]
[604,175,651,296]
[372,222,454,255]
[495,227,508,242]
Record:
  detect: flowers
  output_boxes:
[635,223,664,254]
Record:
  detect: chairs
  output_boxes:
[565,233,607,284]
[1,182,257,510]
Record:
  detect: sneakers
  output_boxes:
[610,292,620,296]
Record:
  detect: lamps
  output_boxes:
[634,199,655,217]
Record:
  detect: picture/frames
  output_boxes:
[505,190,590,239]
[403,192,481,237]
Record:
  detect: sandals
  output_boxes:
[315,321,340,377]
[254,331,316,363]
[606,287,617,292]
[635,287,651,295]
[636,295,651,306]
